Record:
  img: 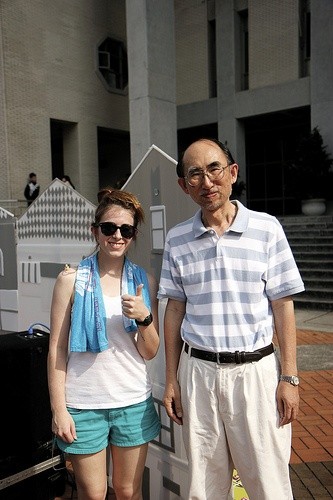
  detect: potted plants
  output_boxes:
[284,125,333,217]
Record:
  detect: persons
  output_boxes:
[62,175,75,190]
[24,172,40,206]
[160,139,305,500]
[47,189,160,500]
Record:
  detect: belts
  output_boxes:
[183,342,273,364]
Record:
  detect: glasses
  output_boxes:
[181,163,230,187]
[91,222,137,239]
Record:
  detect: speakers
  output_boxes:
[0,328,62,481]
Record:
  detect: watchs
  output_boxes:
[280,375,300,386]
[135,313,153,326]
